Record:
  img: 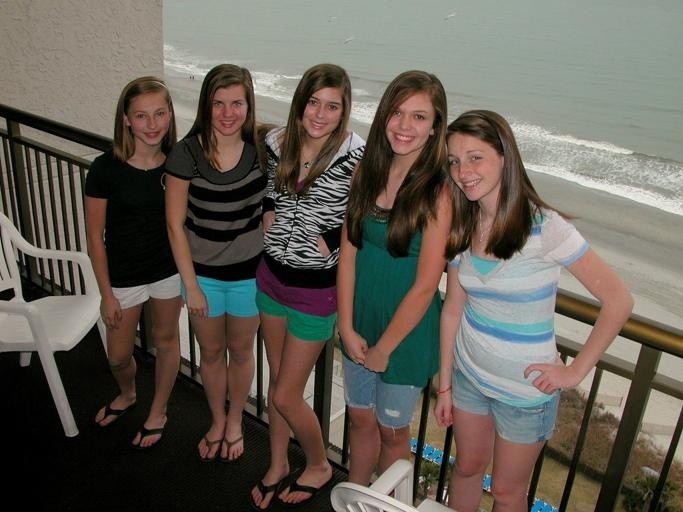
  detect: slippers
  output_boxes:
[96,398,137,427]
[279,470,332,506]
[249,473,291,511]
[133,416,168,449]
[197,436,222,462]
[218,420,246,463]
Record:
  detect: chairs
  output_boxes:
[0,207,111,442]
[329,457,460,512]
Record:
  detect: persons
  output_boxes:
[84,78,183,450]
[434,109,634,512]
[248,64,367,512]
[162,63,278,462]
[335,70,453,499]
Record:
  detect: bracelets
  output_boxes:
[437,385,452,395]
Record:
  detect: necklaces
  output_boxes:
[479,207,492,244]
[300,160,314,168]
[130,160,161,171]
